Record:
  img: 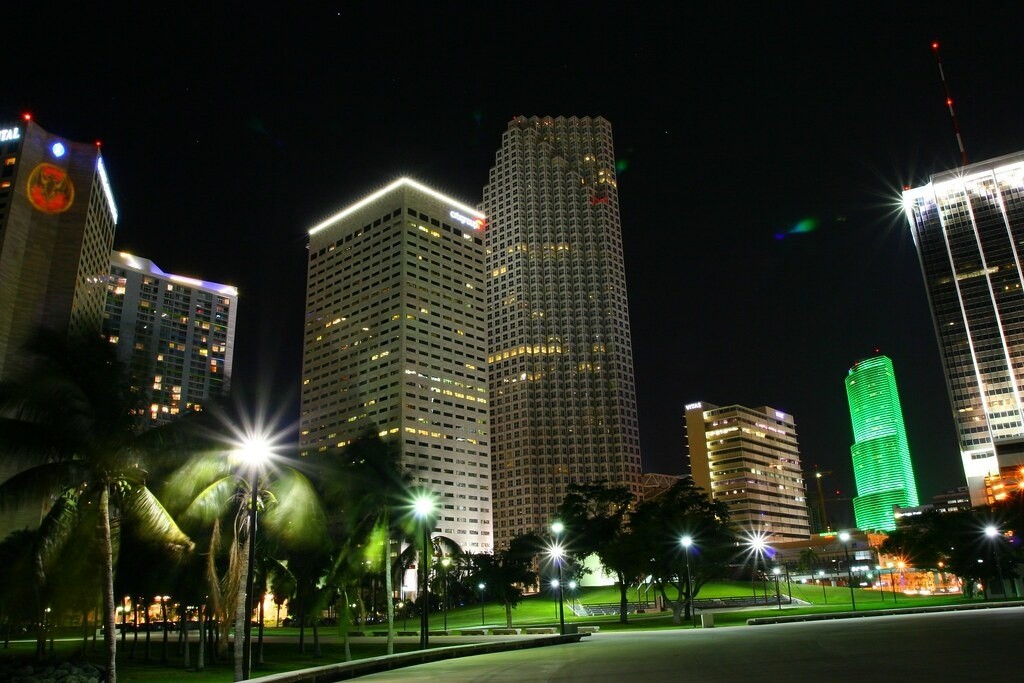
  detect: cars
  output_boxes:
[114,612,385,633]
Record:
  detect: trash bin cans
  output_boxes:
[564,623,578,634]
[701,614,714,627]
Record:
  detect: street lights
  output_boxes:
[772,567,781,609]
[680,536,697,628]
[551,579,560,620]
[875,565,885,601]
[753,539,770,604]
[569,581,576,616]
[479,584,486,625]
[840,531,856,610]
[551,520,565,635]
[234,435,270,680]
[886,562,898,604]
[819,571,828,604]
[412,497,433,649]
[985,525,1008,601]
